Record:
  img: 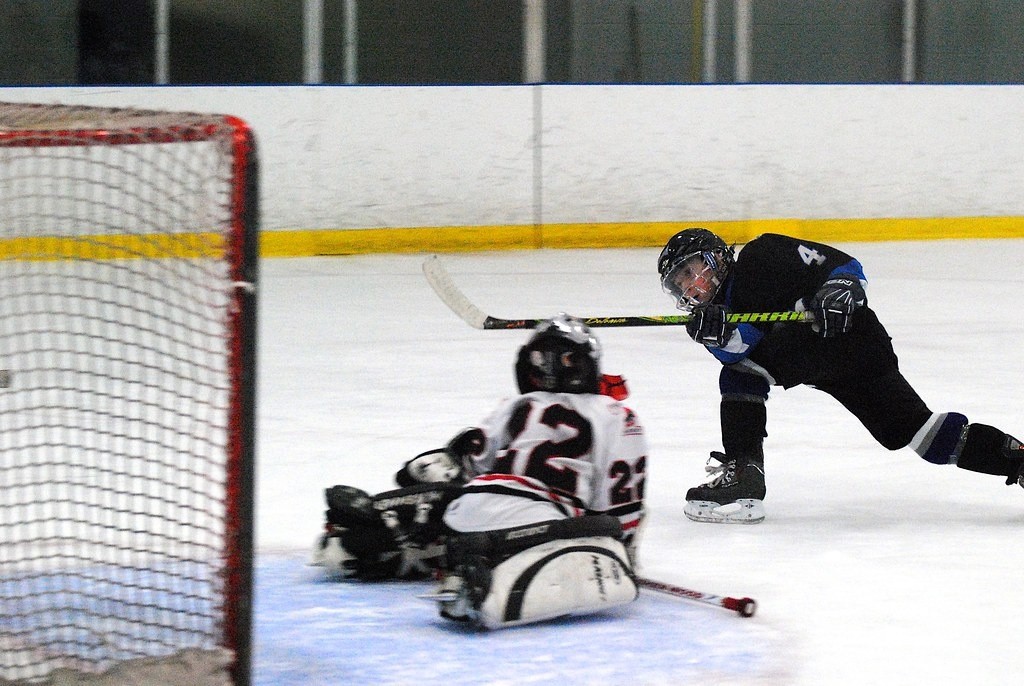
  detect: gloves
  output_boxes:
[687,305,735,349]
[811,277,865,338]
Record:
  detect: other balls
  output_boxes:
[0,370,12,391]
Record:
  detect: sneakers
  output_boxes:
[684,451,767,524]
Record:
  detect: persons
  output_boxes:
[658,227,1024,524]
[325,314,648,631]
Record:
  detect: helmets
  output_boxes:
[656,228,731,288]
[515,316,601,394]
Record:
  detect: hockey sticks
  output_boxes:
[634,574,756,617]
[421,254,818,330]
